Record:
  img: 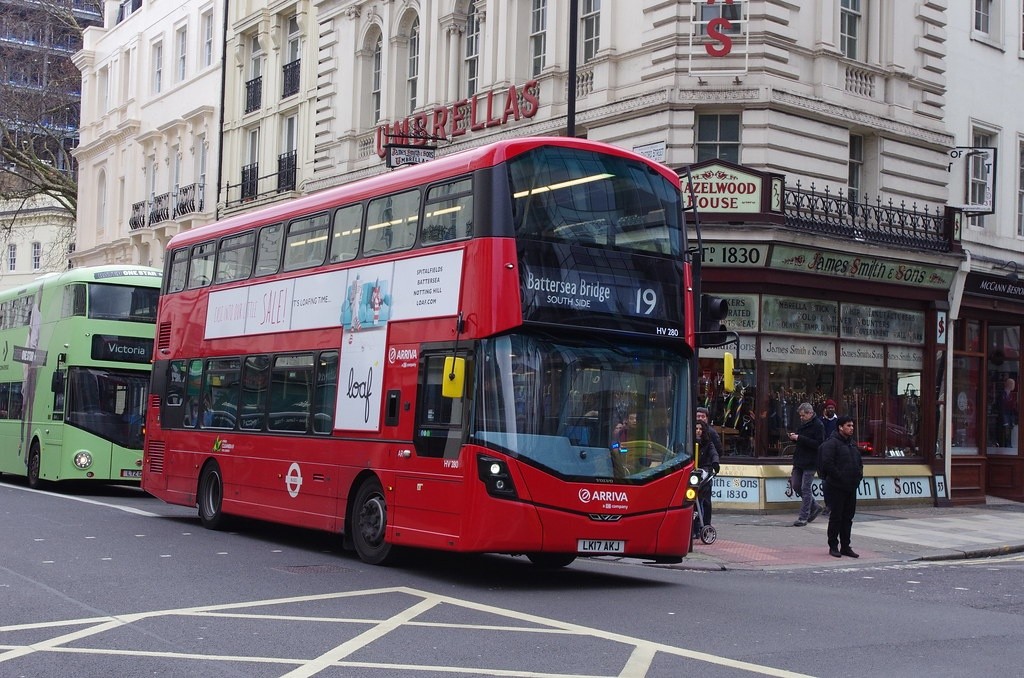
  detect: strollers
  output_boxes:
[692,465,718,545]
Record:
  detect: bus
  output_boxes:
[0,263,163,491]
[136,136,701,582]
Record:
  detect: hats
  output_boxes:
[825,399,837,408]
[697,407,710,417]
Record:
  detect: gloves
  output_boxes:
[713,462,720,473]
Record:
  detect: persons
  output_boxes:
[1001,378,1016,401]
[695,406,724,458]
[616,411,653,467]
[816,419,865,559]
[784,402,826,528]
[691,419,720,527]
[820,400,840,420]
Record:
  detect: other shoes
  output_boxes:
[829,546,841,556]
[840,545,860,558]
[794,518,808,526]
[808,505,823,521]
[823,507,830,515]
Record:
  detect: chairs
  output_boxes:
[173,246,405,294]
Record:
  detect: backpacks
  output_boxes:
[816,437,839,479]
